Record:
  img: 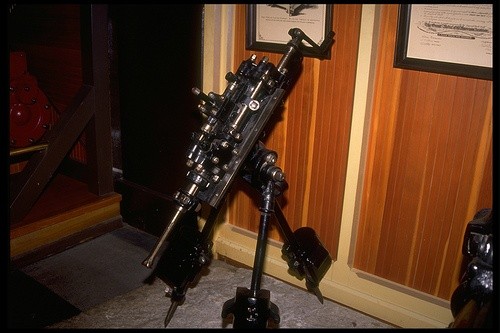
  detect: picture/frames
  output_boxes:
[245,4,331,60]
[392,3,494,81]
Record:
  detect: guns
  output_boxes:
[141,27,333,328]
